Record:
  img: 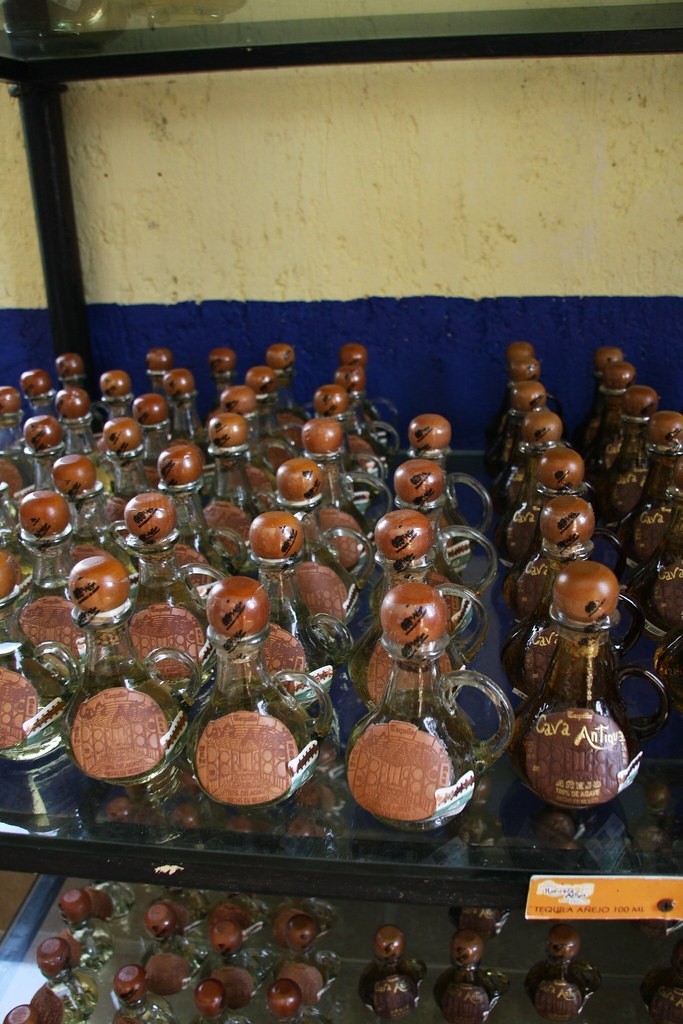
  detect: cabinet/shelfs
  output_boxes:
[0,0,683,1024]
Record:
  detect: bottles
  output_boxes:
[0,341,683,830]
[3,881,683,1024]
